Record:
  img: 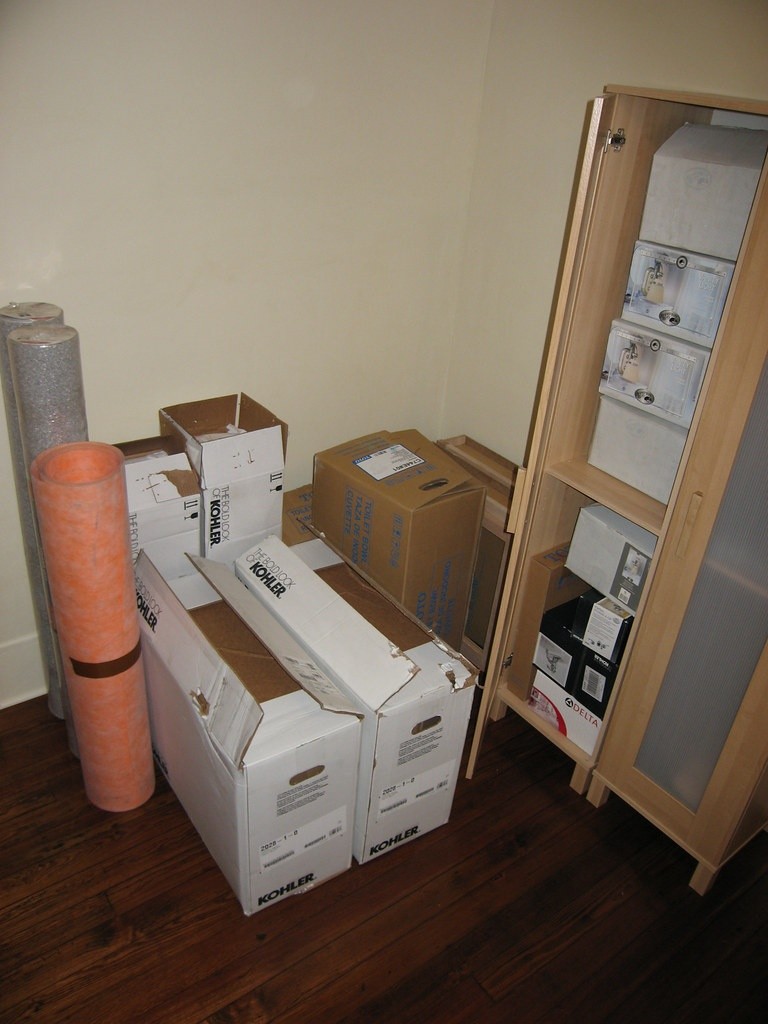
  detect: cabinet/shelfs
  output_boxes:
[464,84,768,897]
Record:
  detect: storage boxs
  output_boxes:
[117,392,525,920]
[527,108,766,758]
